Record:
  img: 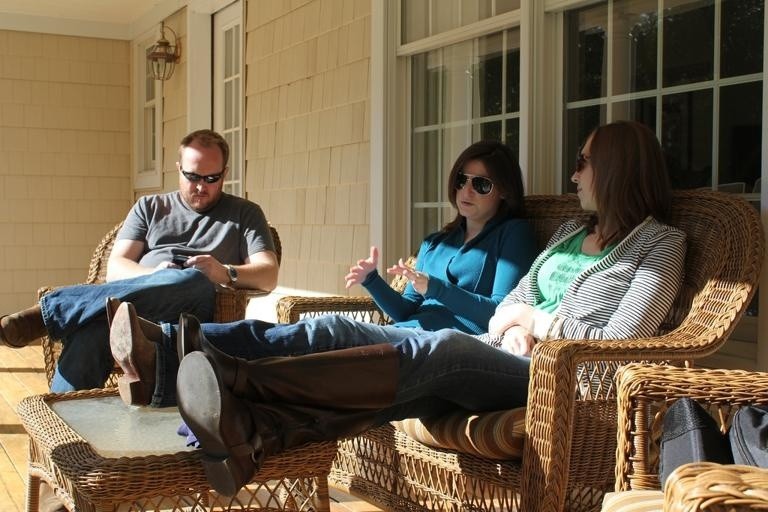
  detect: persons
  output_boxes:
[1,129,279,392]
[175,121,687,496]
[106,139,530,406]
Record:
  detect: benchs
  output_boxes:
[277,188,763,512]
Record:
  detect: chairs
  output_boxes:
[37,217,283,391]
[597,364,768,512]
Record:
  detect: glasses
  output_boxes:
[453,173,497,196]
[180,166,226,183]
[575,153,593,173]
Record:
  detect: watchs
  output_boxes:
[223,264,238,286]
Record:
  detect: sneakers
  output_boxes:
[0,303,49,348]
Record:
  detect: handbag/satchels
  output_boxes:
[658,397,767,494]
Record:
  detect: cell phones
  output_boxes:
[172,255,188,267]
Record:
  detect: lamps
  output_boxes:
[148,20,182,81]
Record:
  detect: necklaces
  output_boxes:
[593,222,619,252]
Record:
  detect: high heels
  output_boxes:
[106,296,162,407]
